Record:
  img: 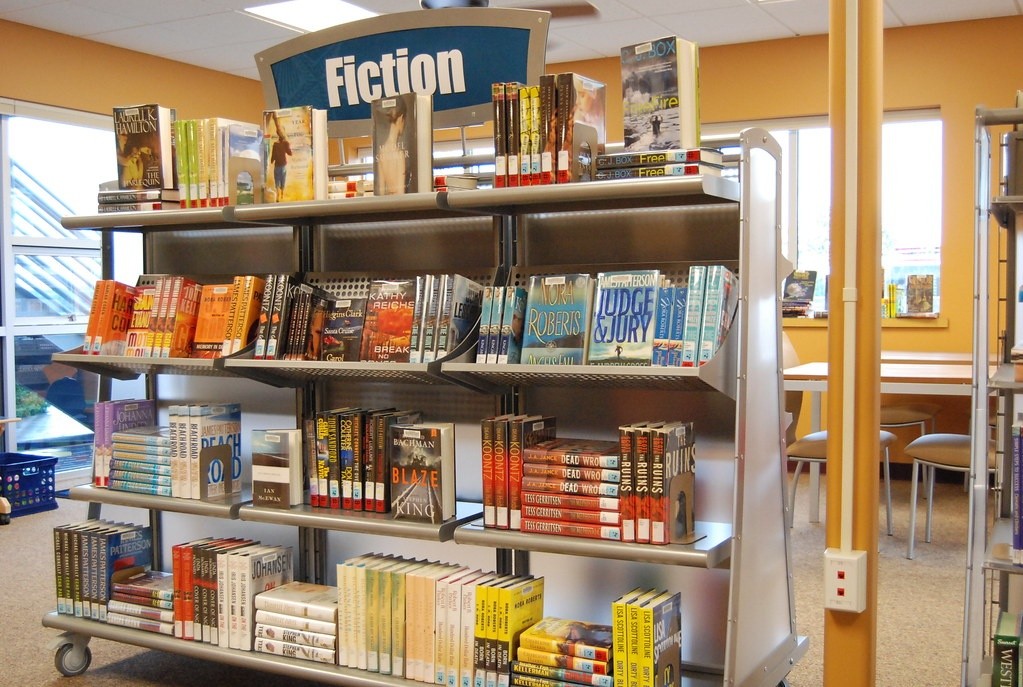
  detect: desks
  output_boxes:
[783,349,1002,521]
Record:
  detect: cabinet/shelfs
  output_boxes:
[962,105,1023,687]
[40,129,801,687]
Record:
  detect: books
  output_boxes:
[80,262,740,380]
[782,269,816,318]
[336,550,682,687]
[174,536,338,669]
[990,127,1022,687]
[97,32,723,213]
[53,517,173,637]
[93,395,242,500]
[249,403,459,525]
[479,410,699,546]
[880,272,935,320]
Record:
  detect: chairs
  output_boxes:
[904,434,996,560]
[782,331,896,536]
[967,404,997,440]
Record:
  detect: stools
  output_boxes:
[880,407,937,499]
